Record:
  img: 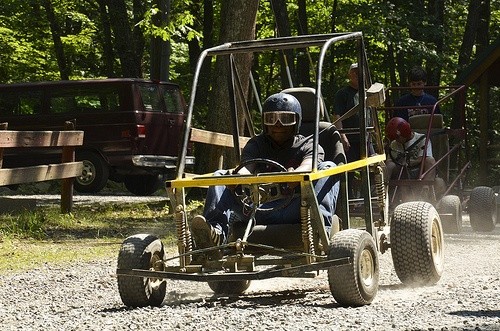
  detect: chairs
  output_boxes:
[230,87,347,248]
[409,113,450,193]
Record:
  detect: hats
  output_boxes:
[348,62,358,75]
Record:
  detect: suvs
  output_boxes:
[0,78,196,196]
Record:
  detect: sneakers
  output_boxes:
[190,214,228,261]
[318,228,331,251]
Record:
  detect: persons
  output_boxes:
[331,63,380,209]
[190,93,340,260]
[393,67,440,122]
[387,117,436,201]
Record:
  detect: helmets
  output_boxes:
[261,92,302,134]
[386,117,412,141]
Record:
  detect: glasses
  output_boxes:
[263,110,297,125]
